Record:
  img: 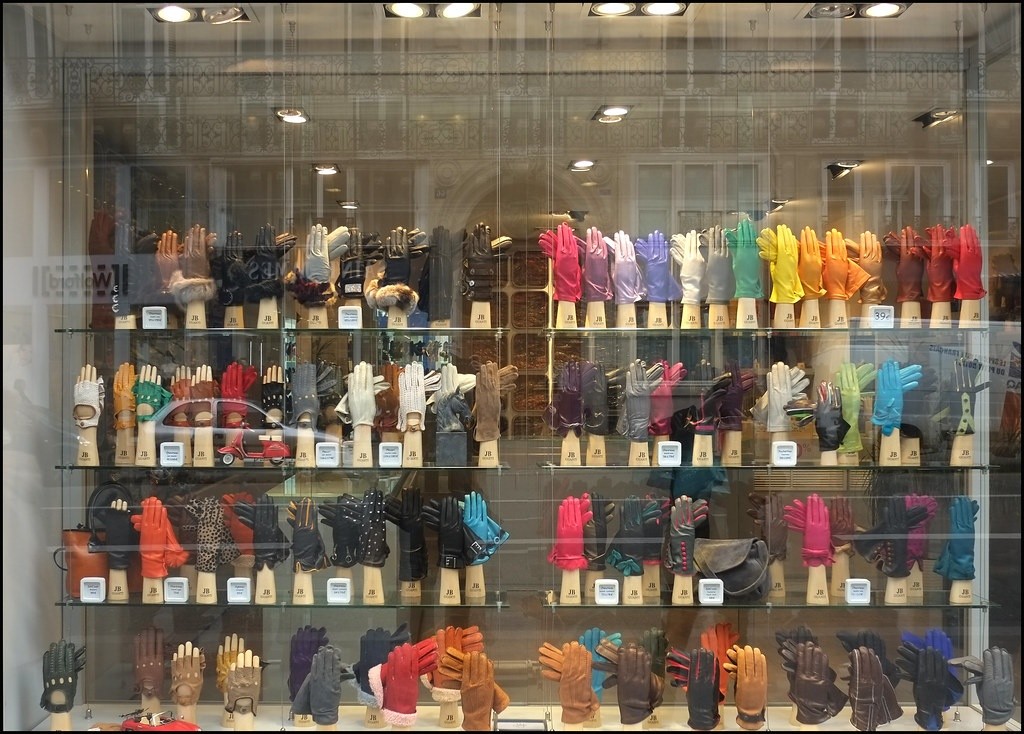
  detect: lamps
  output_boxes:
[569,210,584,222]
[922,113,940,129]
[827,164,850,183]
[200,8,245,25]
[748,210,767,223]
[766,202,783,213]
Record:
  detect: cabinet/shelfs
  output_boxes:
[537,328,995,609]
[55,326,510,608]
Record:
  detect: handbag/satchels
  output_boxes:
[690,538,769,607]
[52,482,143,597]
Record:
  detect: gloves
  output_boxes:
[40,640,87,713]
[129,486,512,578]
[88,208,508,329]
[537,210,1016,730]
[135,626,169,700]
[290,360,516,443]
[222,363,257,426]
[286,623,511,731]
